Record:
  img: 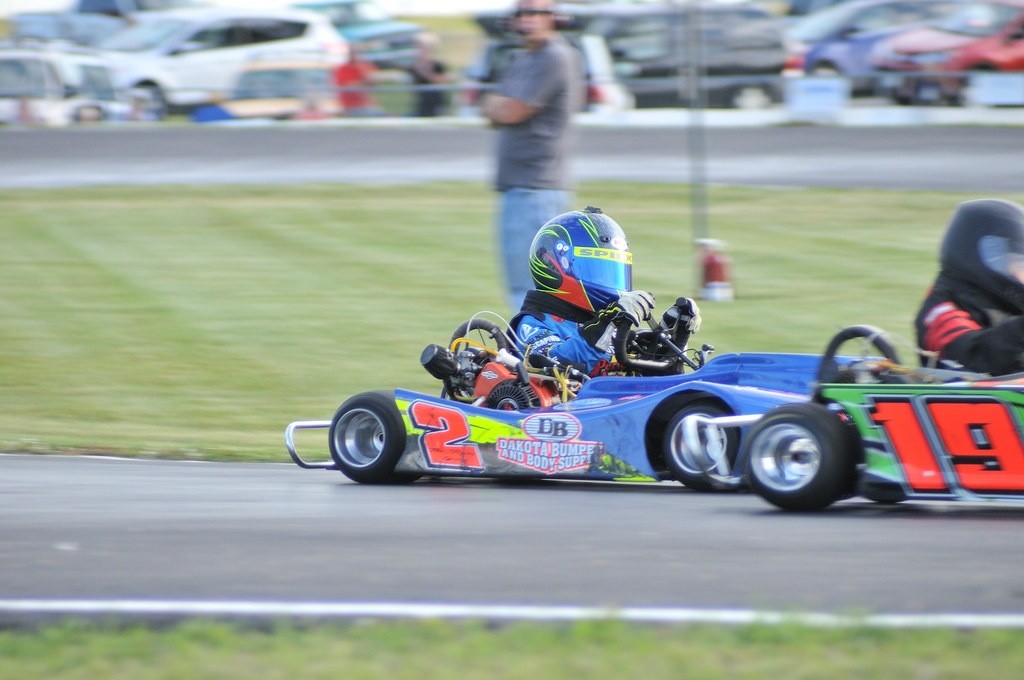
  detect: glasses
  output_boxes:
[514,9,553,18]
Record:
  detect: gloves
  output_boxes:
[578,291,657,354]
[654,298,701,335]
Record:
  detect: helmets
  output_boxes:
[529,206,633,314]
[938,199,1024,312]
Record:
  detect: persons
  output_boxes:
[335,43,382,115]
[480,0,579,311]
[410,36,452,116]
[505,205,702,385]
[914,199,1024,382]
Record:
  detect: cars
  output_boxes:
[468,0,1024,110]
[1,3,456,124]
